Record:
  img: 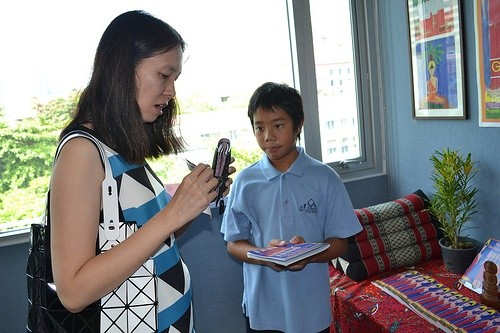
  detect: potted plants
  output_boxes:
[421,147,481,274]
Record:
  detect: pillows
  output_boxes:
[337,189,445,282]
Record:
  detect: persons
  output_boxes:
[220,81,363,333]
[47,11,236,333]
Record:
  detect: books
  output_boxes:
[246,241,330,267]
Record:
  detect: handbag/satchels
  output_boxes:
[26,130,159,333]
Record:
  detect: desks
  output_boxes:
[328,257,500,332]
